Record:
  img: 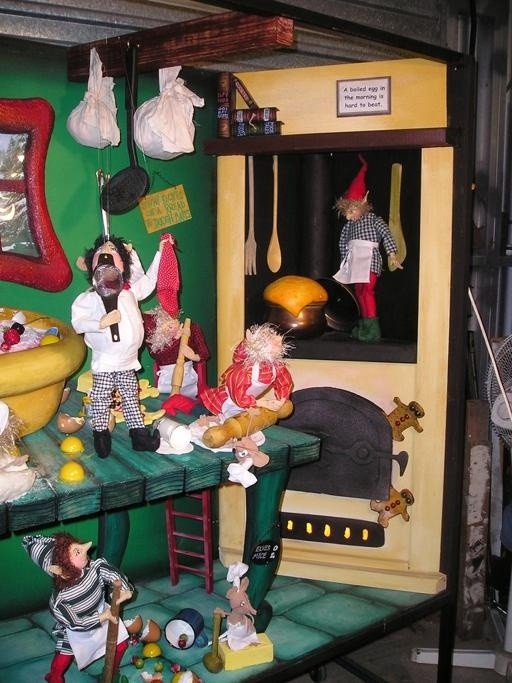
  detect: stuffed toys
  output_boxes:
[1,155,404,682]
[208,561,263,651]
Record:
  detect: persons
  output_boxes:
[72,233,176,459]
[142,302,210,399]
[0,399,60,504]
[331,157,401,342]
[22,532,134,683]
[200,322,295,467]
